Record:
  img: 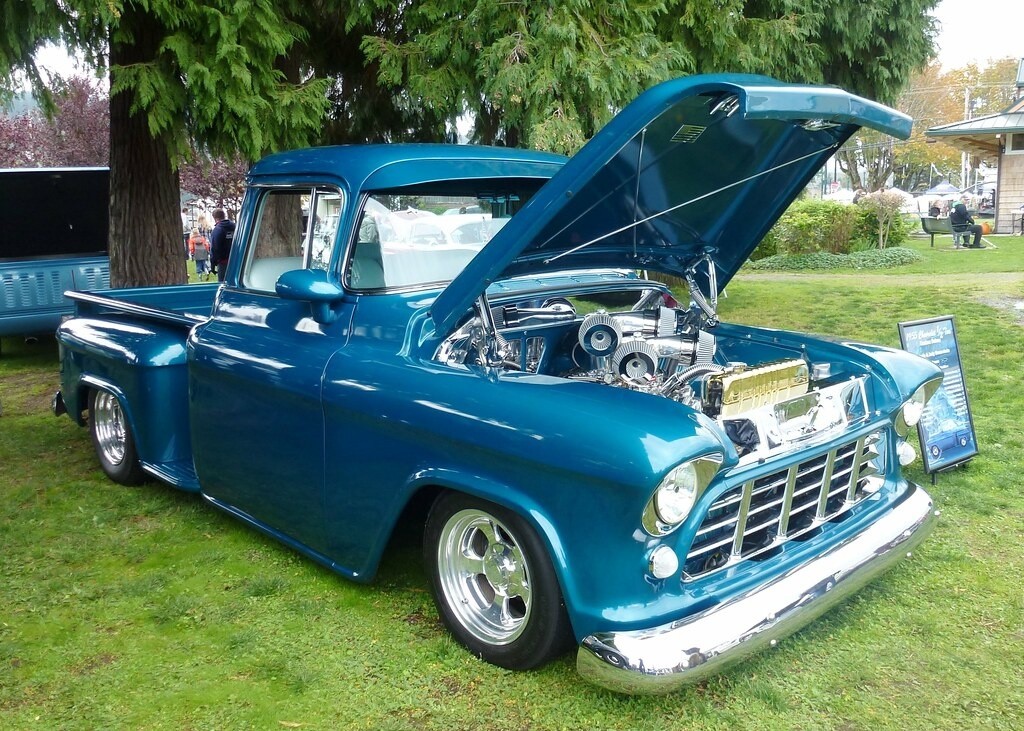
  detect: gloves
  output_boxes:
[211,265,216,275]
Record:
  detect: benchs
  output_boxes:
[920,217,971,249]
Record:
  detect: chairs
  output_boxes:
[246,250,478,293]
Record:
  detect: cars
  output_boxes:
[51,72,950,700]
[297,201,509,270]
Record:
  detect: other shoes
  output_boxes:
[205,268,209,281]
[963,242,970,247]
[198,273,203,282]
[973,244,986,248]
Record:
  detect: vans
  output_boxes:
[0,166,115,350]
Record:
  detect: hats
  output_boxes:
[961,195,970,200]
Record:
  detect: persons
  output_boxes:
[950,195,986,248]
[180,208,191,259]
[210,208,236,282]
[196,214,212,271]
[853,189,862,204]
[990,189,995,207]
[189,228,210,281]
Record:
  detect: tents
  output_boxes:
[925,180,959,194]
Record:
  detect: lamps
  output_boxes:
[995,134,1003,150]
